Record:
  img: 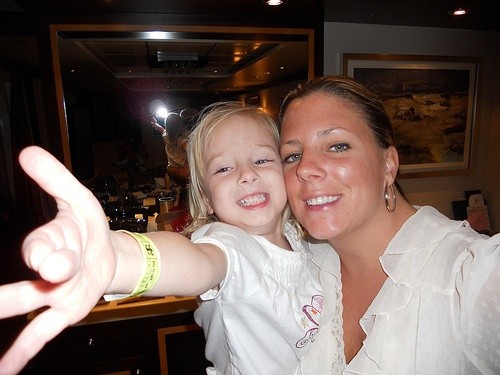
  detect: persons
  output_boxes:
[150,112,197,207]
[0,101,324,375]
[180,107,199,125]
[279,76,500,375]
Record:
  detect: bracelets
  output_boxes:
[103,230,162,303]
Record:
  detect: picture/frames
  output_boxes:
[339,53,485,178]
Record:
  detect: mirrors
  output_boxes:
[48,24,324,233]
